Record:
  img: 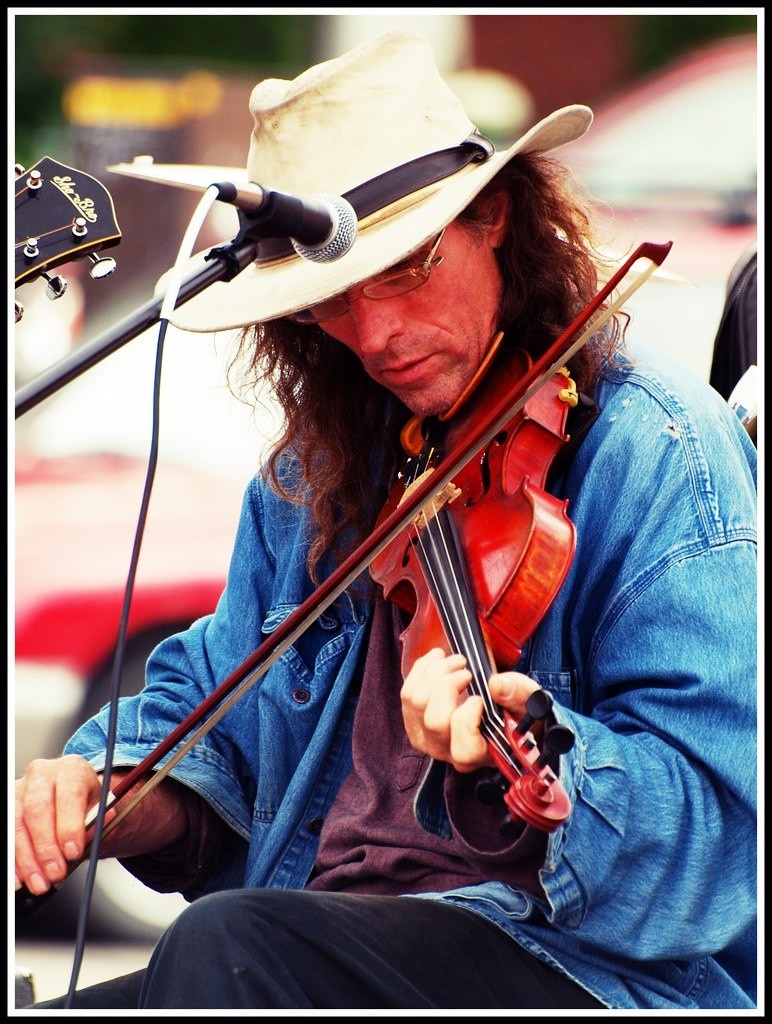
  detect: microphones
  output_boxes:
[214,181,357,263]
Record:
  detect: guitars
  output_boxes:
[15,147,129,328]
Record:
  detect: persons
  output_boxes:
[16,28,759,1010]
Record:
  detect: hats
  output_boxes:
[153,26,593,334]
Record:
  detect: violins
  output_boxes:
[364,342,584,834]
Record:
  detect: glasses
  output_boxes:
[292,229,448,324]
